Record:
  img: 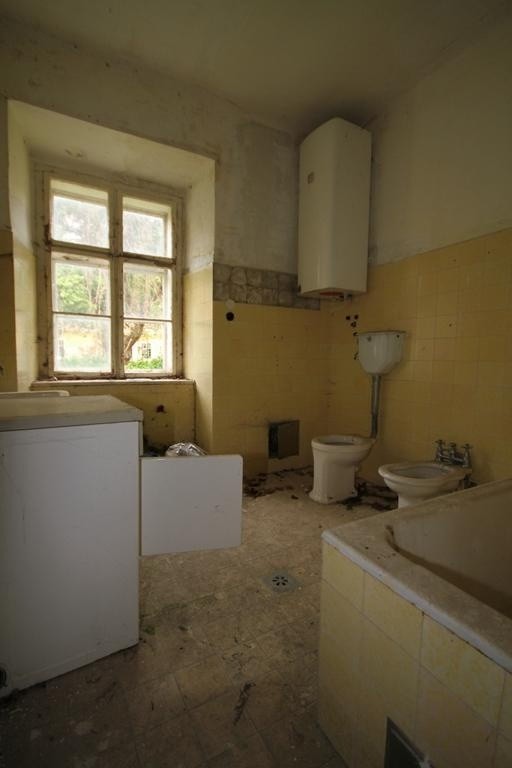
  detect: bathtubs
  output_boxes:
[318,475,512,768]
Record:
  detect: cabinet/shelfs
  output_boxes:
[0,394,244,701]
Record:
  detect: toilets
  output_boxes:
[310,435,375,505]
[378,459,466,509]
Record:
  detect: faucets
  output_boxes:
[438,441,459,463]
